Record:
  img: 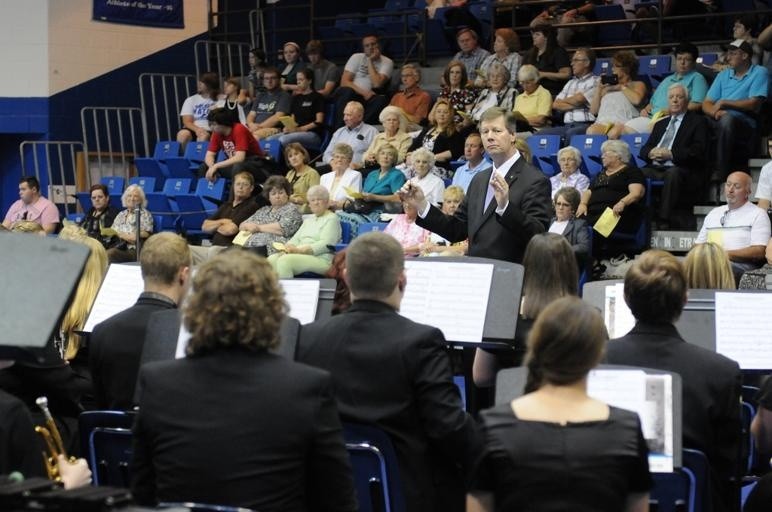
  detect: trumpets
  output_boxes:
[35,396,77,485]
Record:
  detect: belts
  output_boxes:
[564,120,599,125]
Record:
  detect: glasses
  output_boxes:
[262,75,278,82]
[570,57,589,64]
[719,210,729,225]
[555,201,571,208]
[520,79,535,86]
[400,73,413,77]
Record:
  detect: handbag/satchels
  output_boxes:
[102,234,129,252]
[341,197,370,215]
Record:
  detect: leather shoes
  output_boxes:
[656,219,668,228]
[709,171,725,181]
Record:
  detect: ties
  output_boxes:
[659,114,677,151]
[484,171,497,215]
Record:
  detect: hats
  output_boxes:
[723,39,757,61]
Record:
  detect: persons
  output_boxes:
[234,178,300,259]
[702,15,759,84]
[346,146,407,215]
[465,63,515,122]
[473,231,579,389]
[300,231,477,505]
[535,50,599,142]
[703,40,769,170]
[407,97,465,165]
[603,0,644,28]
[363,108,412,165]
[409,184,474,257]
[446,134,491,196]
[575,140,644,252]
[247,70,290,140]
[471,296,652,512]
[585,51,645,143]
[250,48,274,97]
[177,74,221,155]
[263,185,340,281]
[438,30,491,87]
[509,65,550,128]
[19,236,109,407]
[474,29,524,87]
[541,187,588,256]
[429,65,479,126]
[278,42,308,93]
[390,65,431,125]
[548,145,589,193]
[326,100,377,172]
[696,172,769,283]
[109,184,154,264]
[77,184,122,251]
[340,34,394,131]
[399,109,550,262]
[642,83,707,230]
[386,149,444,208]
[313,144,364,211]
[282,140,321,216]
[625,44,706,134]
[678,241,736,291]
[2,176,59,238]
[194,108,271,184]
[382,186,428,260]
[267,65,324,161]
[91,231,194,412]
[530,0,599,47]
[135,251,358,512]
[205,75,246,130]
[592,250,742,512]
[516,24,571,131]
[303,43,338,99]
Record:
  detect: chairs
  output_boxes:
[697,53,717,66]
[134,141,181,181]
[737,384,766,485]
[110,177,156,213]
[634,177,652,257]
[67,214,85,223]
[216,150,228,163]
[296,0,494,61]
[577,223,593,292]
[335,222,390,252]
[639,53,671,91]
[592,57,614,77]
[620,133,651,170]
[152,215,161,235]
[79,406,141,491]
[76,175,125,214]
[176,177,227,236]
[346,439,390,512]
[553,133,609,179]
[525,133,562,177]
[326,221,351,257]
[260,139,281,164]
[648,447,731,511]
[164,141,211,179]
[450,148,495,168]
[144,178,192,229]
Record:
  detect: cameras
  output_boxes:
[93,232,131,251]
[600,73,620,87]
[342,197,385,217]
